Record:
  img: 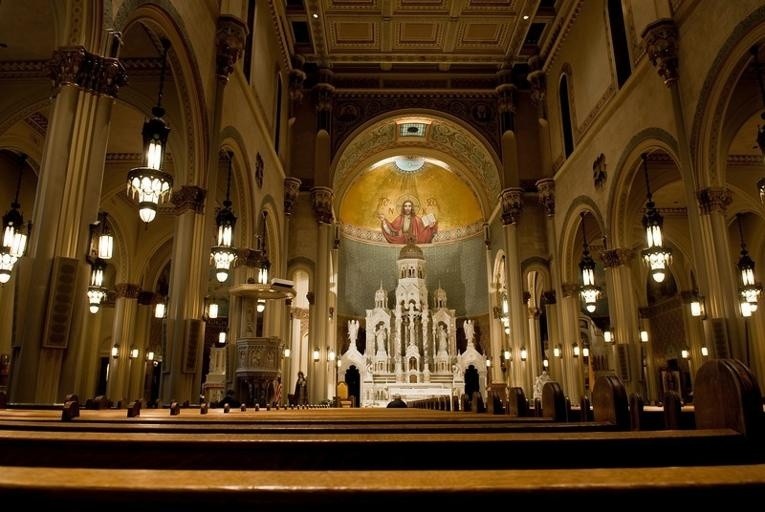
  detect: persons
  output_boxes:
[348,319,359,348]
[217,390,241,408]
[463,319,476,349]
[276,377,282,406]
[294,371,308,405]
[436,325,448,357]
[268,379,277,404]
[374,325,387,356]
[385,395,407,408]
[377,200,440,244]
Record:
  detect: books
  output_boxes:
[422,213,438,228]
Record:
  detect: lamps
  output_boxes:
[209,147,242,283]
[553,343,563,360]
[129,343,138,359]
[734,211,764,314]
[500,254,511,334]
[502,347,514,361]
[111,343,119,360]
[90,211,114,260]
[218,329,229,345]
[639,329,649,345]
[126,34,175,224]
[144,345,154,362]
[603,327,616,345]
[638,150,674,284]
[700,344,710,360]
[10,219,31,257]
[326,345,335,362]
[336,356,342,367]
[0,152,28,285]
[688,295,707,321]
[202,294,218,321]
[312,344,321,362]
[256,209,272,313]
[574,210,602,313]
[571,343,580,358]
[518,344,529,361]
[735,293,752,320]
[681,346,693,360]
[582,344,590,358]
[86,212,109,314]
[153,299,168,319]
[282,342,290,359]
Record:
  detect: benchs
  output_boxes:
[0,360,765,512]
